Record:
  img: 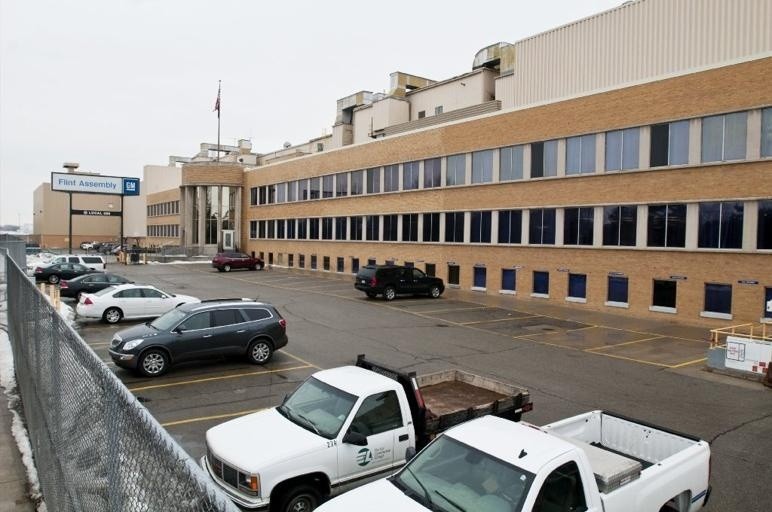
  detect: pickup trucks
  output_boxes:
[308,409,712,512]
[201,354,536,512]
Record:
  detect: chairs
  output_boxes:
[537,470,576,512]
[363,397,395,424]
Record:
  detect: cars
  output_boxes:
[354,264,445,301]
[80,241,131,255]
[26,255,288,377]
[212,252,265,272]
